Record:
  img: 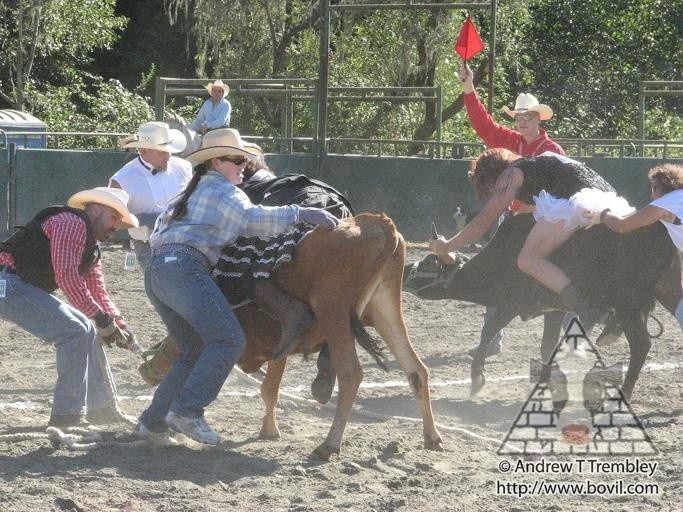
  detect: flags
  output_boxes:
[454,16,485,62]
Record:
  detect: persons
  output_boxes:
[0,63,683,448]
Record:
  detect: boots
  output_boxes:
[595,312,625,347]
[555,283,608,337]
[311,346,337,405]
[251,278,315,363]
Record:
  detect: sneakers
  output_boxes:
[132,422,180,446]
[47,413,92,430]
[84,403,136,430]
[164,409,221,445]
[469,342,499,356]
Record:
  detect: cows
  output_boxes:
[401,209,683,410]
[137,212,447,462]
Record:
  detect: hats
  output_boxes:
[66,186,140,228]
[502,93,553,121]
[207,78,230,98]
[120,121,187,154]
[184,128,265,162]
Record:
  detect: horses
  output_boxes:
[163,109,264,158]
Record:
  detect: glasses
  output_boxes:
[218,156,247,166]
[511,113,538,121]
[651,182,666,193]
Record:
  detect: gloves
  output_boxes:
[94,311,138,352]
[299,207,340,230]
